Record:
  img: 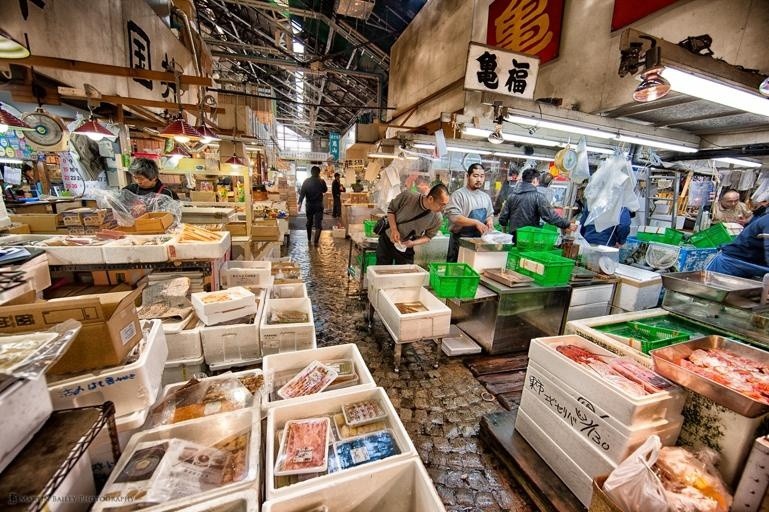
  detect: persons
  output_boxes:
[579,207,631,248]
[443,163,494,263]
[709,189,755,226]
[498,168,577,245]
[708,213,769,280]
[298,166,328,245]
[21,164,35,185]
[332,173,347,218]
[376,183,450,263]
[121,157,179,213]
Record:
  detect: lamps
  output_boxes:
[156,112,204,143]
[632,54,769,120]
[225,156,244,168]
[197,122,216,144]
[409,140,555,163]
[0,108,36,133]
[488,115,506,143]
[621,44,671,104]
[0,29,30,60]
[73,104,113,141]
[459,124,629,156]
[503,113,699,154]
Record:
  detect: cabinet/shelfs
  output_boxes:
[447,272,570,354]
[571,266,617,317]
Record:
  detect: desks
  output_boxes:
[368,303,463,373]
[345,239,379,299]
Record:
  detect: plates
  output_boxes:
[323,371,359,390]
[333,414,387,441]
[274,417,331,477]
[277,359,338,404]
[341,399,388,427]
[649,333,769,417]
[322,358,356,380]
[277,427,336,480]
[333,427,404,472]
[660,270,766,303]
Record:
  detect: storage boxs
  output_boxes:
[524,359,685,464]
[581,245,620,271]
[103,234,175,264]
[690,223,732,248]
[39,234,103,264]
[594,321,688,357]
[649,214,685,230]
[259,341,376,411]
[429,260,479,298]
[264,386,416,501]
[162,357,202,386]
[145,369,261,431]
[570,284,613,306]
[29,436,97,512]
[357,253,376,273]
[332,226,345,238]
[363,220,379,237]
[200,290,265,365]
[367,281,378,309]
[517,250,574,286]
[260,455,444,512]
[0,373,54,474]
[519,389,618,478]
[89,408,259,512]
[112,226,165,234]
[0,283,147,370]
[227,221,278,242]
[355,267,368,289]
[513,408,591,508]
[378,288,451,340]
[164,320,202,360]
[357,252,378,272]
[261,298,315,357]
[20,254,53,292]
[12,212,58,232]
[636,228,684,245]
[87,406,150,463]
[168,230,231,259]
[528,334,686,426]
[135,212,173,231]
[0,234,56,259]
[267,283,306,299]
[46,318,169,416]
[614,263,662,311]
[517,227,559,251]
[567,302,608,320]
[219,261,271,288]
[367,264,429,287]
[355,266,368,287]
[457,247,508,273]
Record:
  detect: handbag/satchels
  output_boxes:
[373,208,430,235]
